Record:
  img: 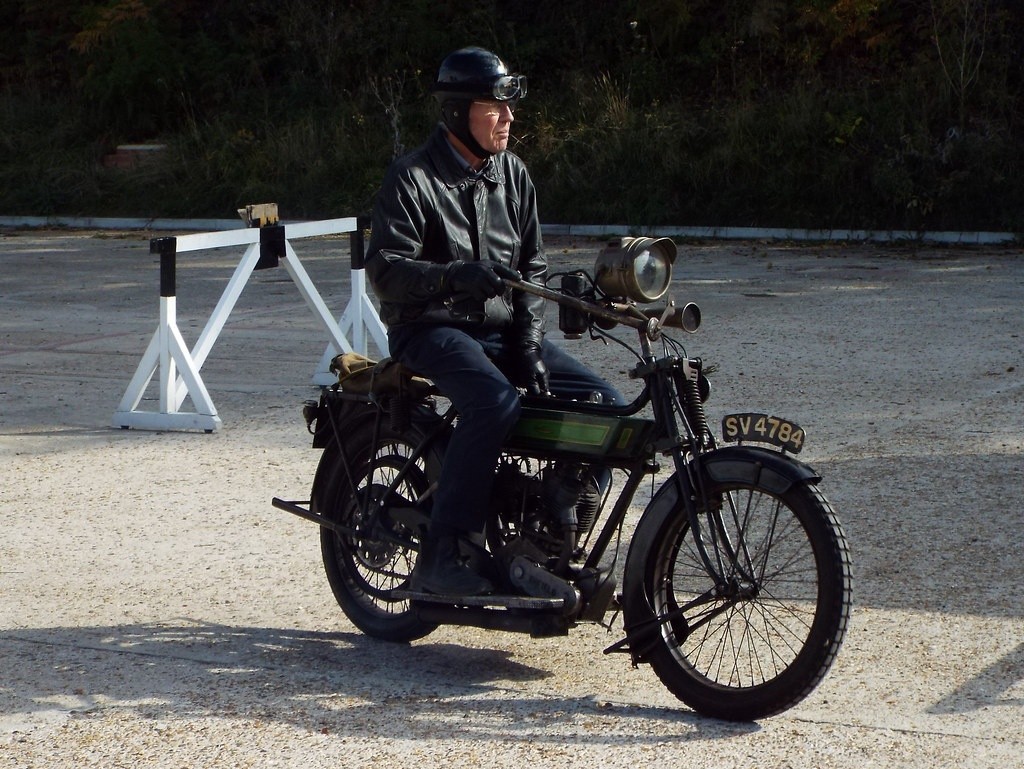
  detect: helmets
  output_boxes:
[435,45,508,105]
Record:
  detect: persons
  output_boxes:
[363,46,631,597]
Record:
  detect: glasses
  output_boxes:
[472,100,517,115]
[484,70,529,101]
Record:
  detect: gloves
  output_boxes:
[516,346,550,395]
[449,259,522,300]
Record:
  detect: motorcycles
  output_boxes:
[271,233,855,722]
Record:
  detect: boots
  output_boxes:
[405,522,496,597]
